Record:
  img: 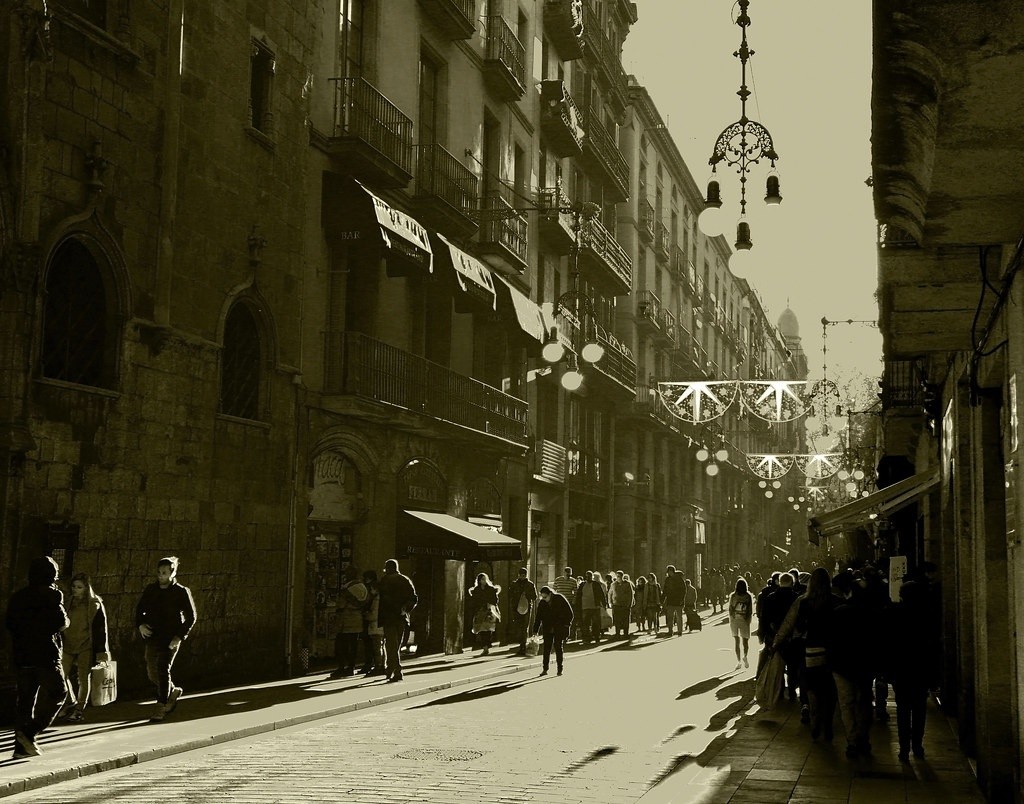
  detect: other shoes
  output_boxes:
[330,668,354,678]
[799,695,927,761]
[540,671,548,676]
[383,667,403,682]
[367,667,385,676]
[482,647,490,656]
[557,671,562,675]
[357,665,371,675]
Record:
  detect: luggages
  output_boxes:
[689,609,702,632]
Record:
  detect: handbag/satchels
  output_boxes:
[517,594,529,614]
[90,661,120,705]
[524,637,539,656]
[600,606,614,629]
[757,653,784,711]
[471,603,502,635]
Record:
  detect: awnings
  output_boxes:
[322,170,546,347]
[395,507,522,561]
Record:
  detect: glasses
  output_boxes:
[543,594,549,599]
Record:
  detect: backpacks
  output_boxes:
[731,593,752,622]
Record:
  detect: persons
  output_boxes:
[729,579,752,669]
[8,556,71,758]
[755,567,948,761]
[575,570,697,638]
[58,572,112,722]
[136,557,197,722]
[701,567,753,614]
[468,573,501,656]
[554,567,578,641]
[642,573,662,634]
[577,571,606,646]
[509,568,538,655]
[330,566,367,678]
[357,569,387,676]
[533,586,574,676]
[608,570,633,640]
[661,565,686,637]
[379,559,418,681]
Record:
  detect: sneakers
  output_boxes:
[736,656,750,669]
[151,688,183,721]
[12,728,42,759]
[58,707,84,721]
